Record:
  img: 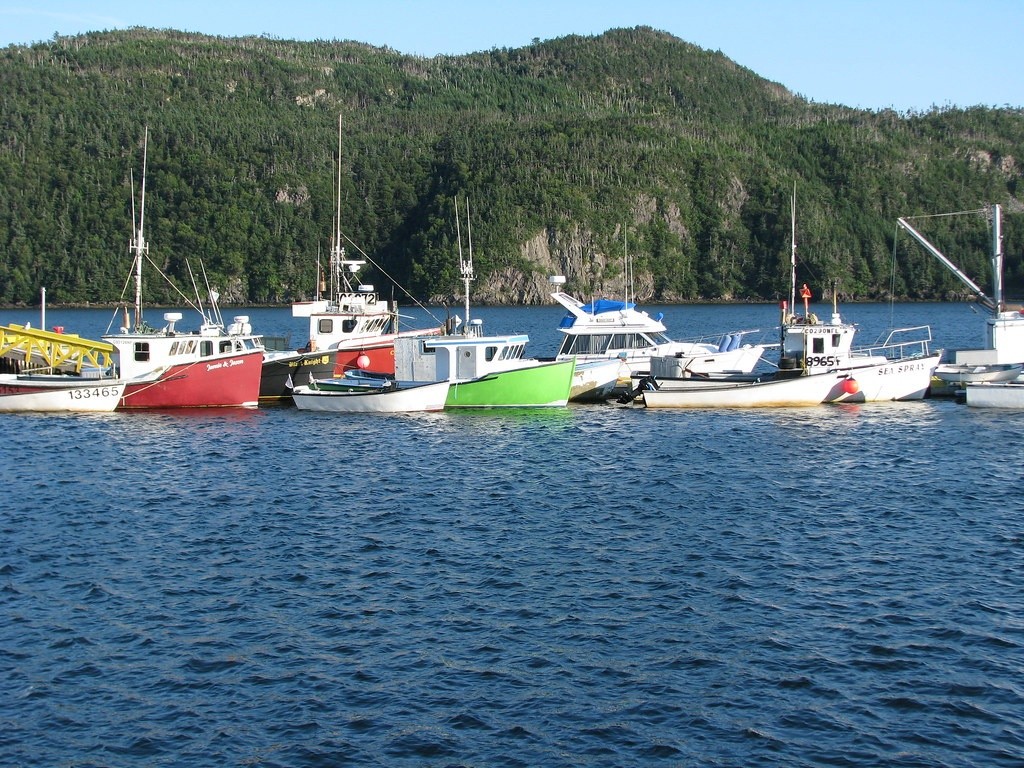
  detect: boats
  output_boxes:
[0,285,128,412]
[890,205,1024,409]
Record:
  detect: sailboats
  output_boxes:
[101,111,578,409]
[550,181,946,407]
